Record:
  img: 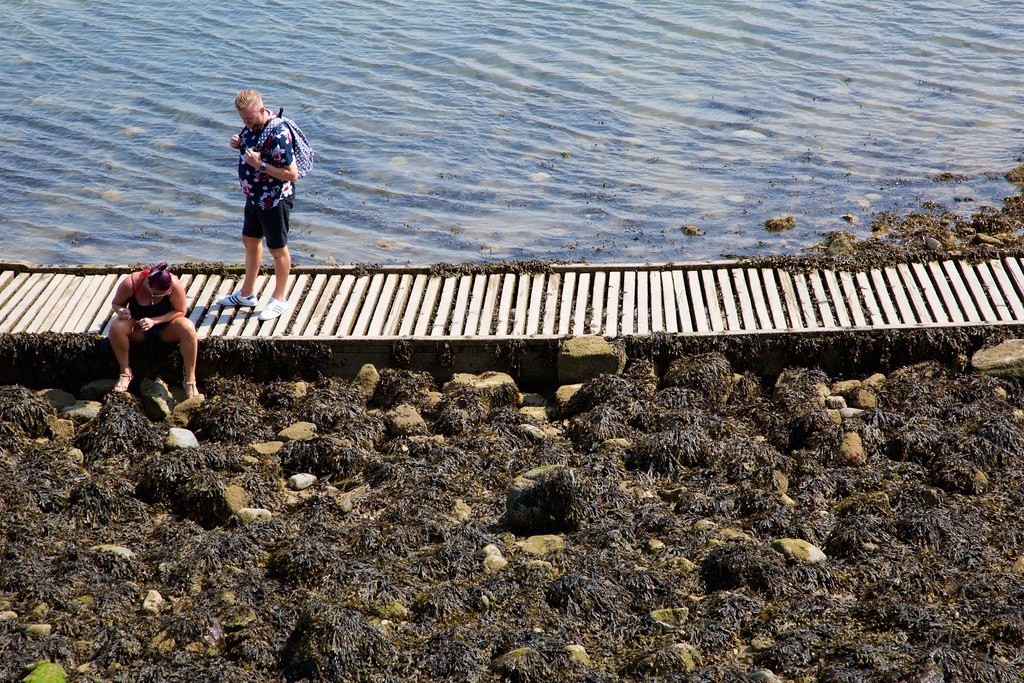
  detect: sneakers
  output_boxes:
[258,297,287,320]
[218,290,258,307]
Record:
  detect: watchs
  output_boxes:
[259,163,266,172]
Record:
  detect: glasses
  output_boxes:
[150,288,171,297]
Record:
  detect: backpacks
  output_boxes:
[241,108,314,179]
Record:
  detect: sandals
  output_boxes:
[114,372,134,392]
[183,377,199,399]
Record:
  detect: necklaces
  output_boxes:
[147,285,171,306]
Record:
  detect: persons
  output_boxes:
[109,262,199,400]
[218,88,298,320]
